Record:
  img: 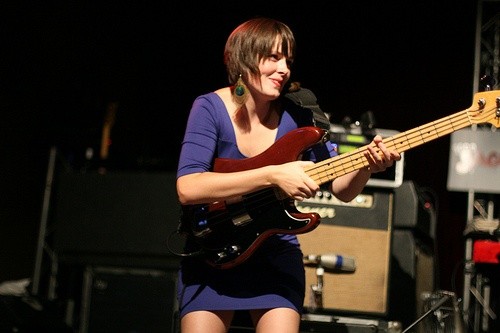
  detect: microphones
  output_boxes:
[302,254,356,274]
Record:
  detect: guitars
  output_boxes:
[181,85,500,269]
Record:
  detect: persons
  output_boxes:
[177,20,401,333]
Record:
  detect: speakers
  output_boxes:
[52,171,187,270]
[294,187,394,315]
